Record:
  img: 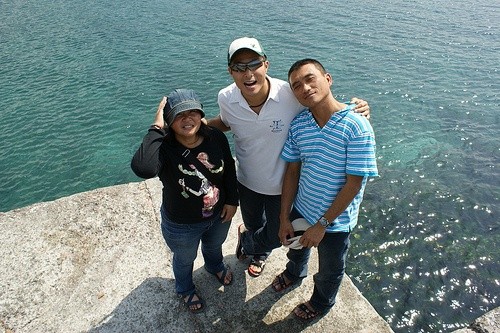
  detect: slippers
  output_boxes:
[248,257,266,276]
[235,224,249,261]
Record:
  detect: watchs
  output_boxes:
[319,217,330,227]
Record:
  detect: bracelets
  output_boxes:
[151,124,162,130]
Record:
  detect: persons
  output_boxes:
[272,58,380,321]
[130,89,239,312]
[200,37,371,277]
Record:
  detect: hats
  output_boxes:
[281,218,311,250]
[228,36,267,65]
[164,88,205,126]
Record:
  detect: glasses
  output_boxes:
[230,58,267,72]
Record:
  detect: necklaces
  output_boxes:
[185,138,200,145]
[251,97,269,108]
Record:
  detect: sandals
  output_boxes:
[271,274,295,291]
[178,291,205,313]
[294,302,320,321]
[204,265,234,285]
